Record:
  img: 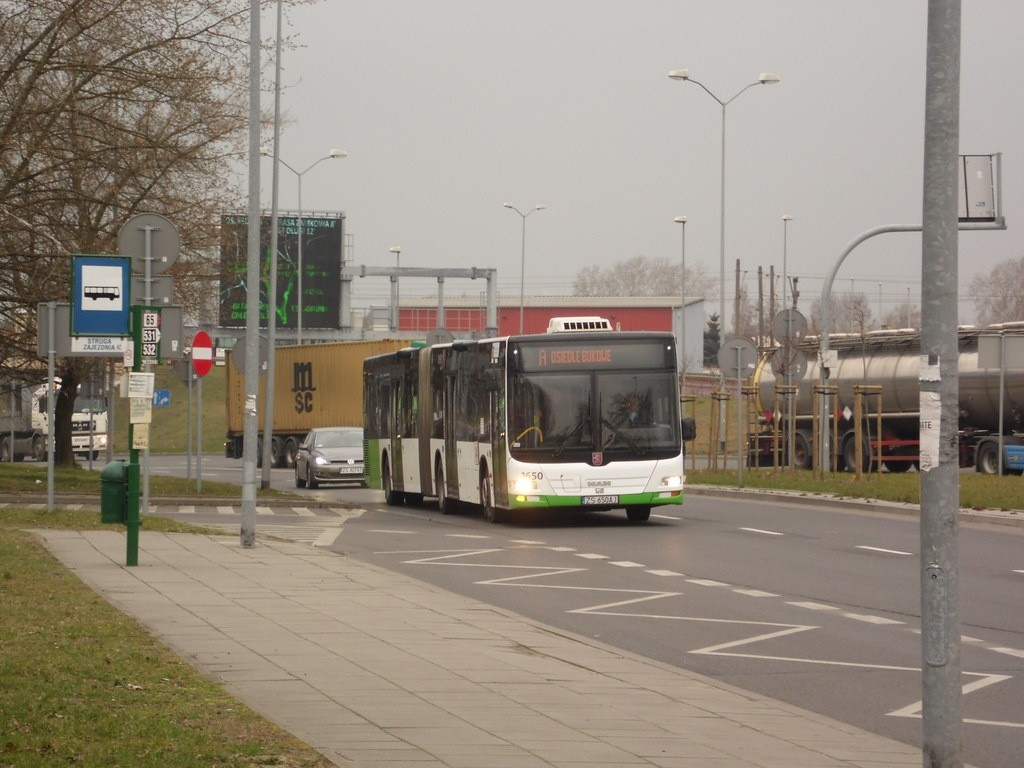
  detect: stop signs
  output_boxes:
[190,330,213,378]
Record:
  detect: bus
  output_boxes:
[362,317,696,525]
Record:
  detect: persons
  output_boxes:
[617,393,656,428]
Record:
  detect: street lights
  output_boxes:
[387,246,400,311]
[257,146,348,346]
[780,213,794,311]
[674,215,688,415]
[668,68,780,443]
[502,203,546,334]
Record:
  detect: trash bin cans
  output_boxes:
[100,460,130,524]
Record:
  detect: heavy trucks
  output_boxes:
[225,336,428,470]
[0,364,108,463]
[747,320,1024,475]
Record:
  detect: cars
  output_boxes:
[295,426,364,489]
